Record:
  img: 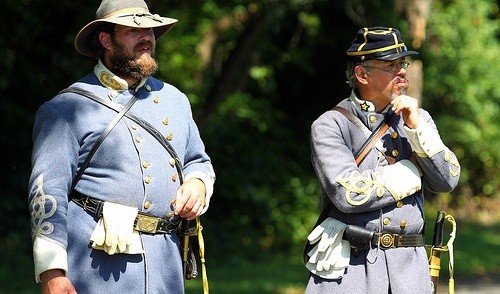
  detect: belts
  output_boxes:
[71,190,177,234]
[372,234,427,250]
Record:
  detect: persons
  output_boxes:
[29,0,217,294]
[303,26,461,294]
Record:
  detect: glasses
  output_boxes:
[365,61,410,72]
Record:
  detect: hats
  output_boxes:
[75,0,178,56]
[345,27,419,65]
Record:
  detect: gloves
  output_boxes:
[89,200,144,255]
[303,218,351,279]
